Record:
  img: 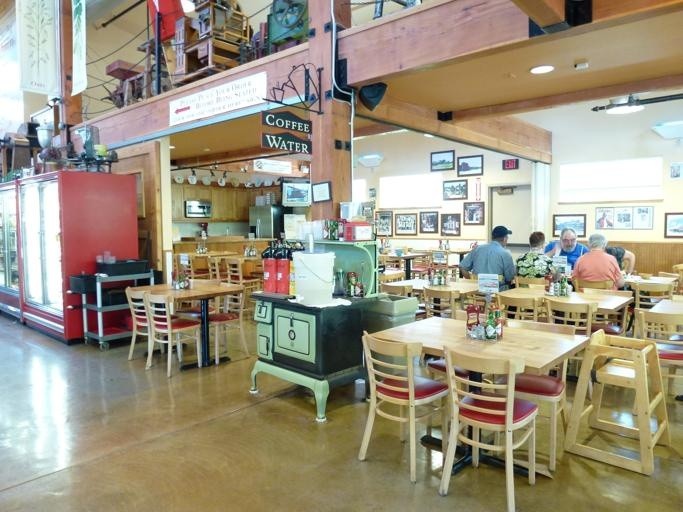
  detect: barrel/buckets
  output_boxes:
[293,250,336,307]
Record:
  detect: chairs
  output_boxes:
[123,287,179,361]
[175,253,206,281]
[224,257,260,307]
[564,329,671,476]
[357,329,448,481]
[423,287,454,317]
[197,284,250,366]
[542,297,597,400]
[574,279,614,291]
[380,282,413,297]
[575,288,633,336]
[633,306,683,417]
[410,248,432,279]
[172,278,220,363]
[438,342,538,512]
[206,254,227,282]
[495,322,576,471]
[494,292,537,323]
[143,292,200,378]
[513,276,544,289]
[426,309,485,386]
[633,282,673,336]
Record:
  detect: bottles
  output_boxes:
[549,282,554,295]
[336,268,345,293]
[485,312,496,339]
[355,283,363,297]
[494,310,503,337]
[347,271,356,296]
[554,283,560,295]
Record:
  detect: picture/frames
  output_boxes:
[462,201,485,226]
[374,210,393,238]
[663,212,683,238]
[439,212,461,237]
[418,211,439,234]
[429,149,455,172]
[456,154,483,178]
[551,213,587,237]
[441,179,468,201]
[394,213,417,236]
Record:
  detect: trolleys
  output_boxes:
[82,269,155,352]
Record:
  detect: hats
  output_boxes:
[492,225,513,239]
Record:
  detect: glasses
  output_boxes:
[561,237,577,242]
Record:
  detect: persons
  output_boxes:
[516,231,551,262]
[545,228,588,269]
[572,234,625,291]
[458,225,517,319]
[604,247,636,275]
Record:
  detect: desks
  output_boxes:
[433,247,473,277]
[623,275,677,283]
[185,251,238,258]
[649,298,683,314]
[368,315,590,475]
[381,250,431,279]
[125,283,243,372]
[380,278,479,296]
[211,255,262,262]
[489,287,635,312]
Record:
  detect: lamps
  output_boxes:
[605,95,645,115]
